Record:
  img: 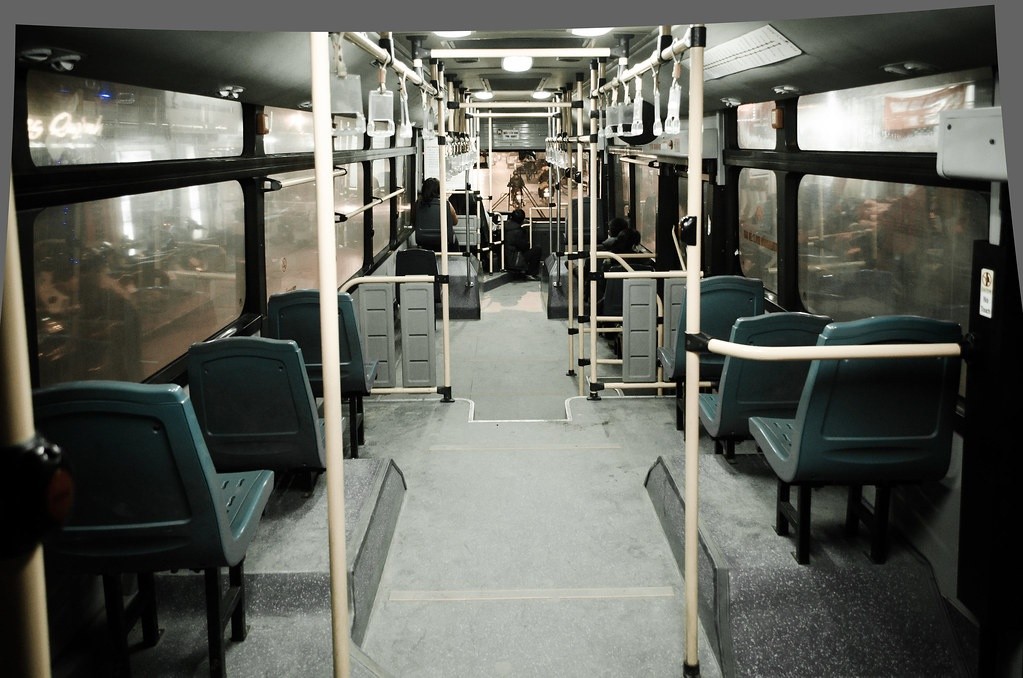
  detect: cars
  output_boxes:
[505,152,523,173]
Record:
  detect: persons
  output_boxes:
[522,151,554,199]
[564,217,655,352]
[410,177,461,252]
[504,208,542,281]
[507,171,525,207]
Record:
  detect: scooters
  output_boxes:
[538,178,555,205]
[507,184,526,210]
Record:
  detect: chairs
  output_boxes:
[267,289,380,460]
[696,311,835,464]
[563,197,605,245]
[748,314,963,565]
[394,248,442,332]
[658,275,765,441]
[414,198,458,252]
[603,265,654,359]
[449,189,490,249]
[187,337,346,498]
[30,379,275,678]
